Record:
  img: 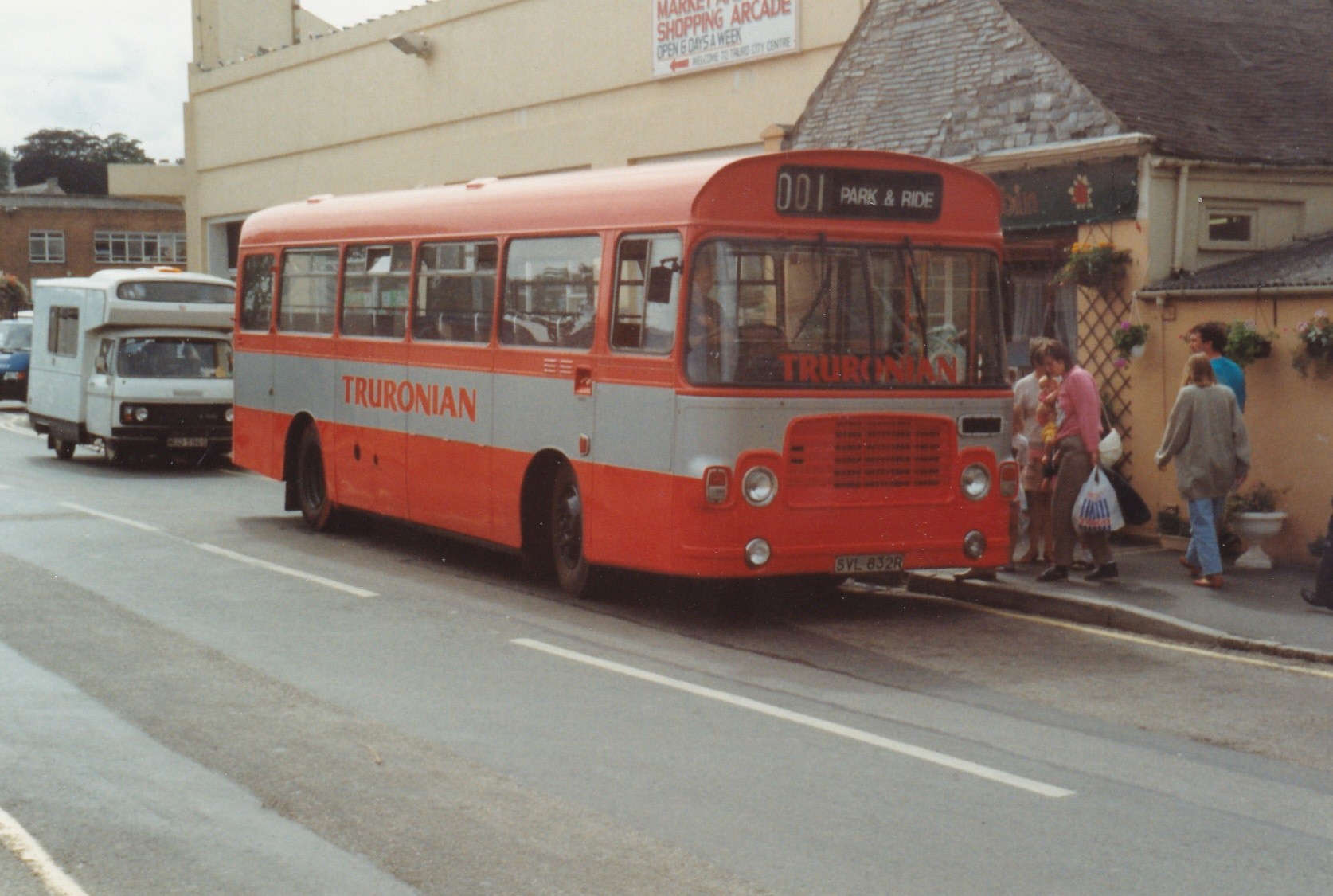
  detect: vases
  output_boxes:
[1073,264,1105,286]
[1304,338,1333,360]
[1128,341,1146,360]
[1250,342,1271,357]
[1231,507,1288,570]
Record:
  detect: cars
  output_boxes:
[0,309,31,402]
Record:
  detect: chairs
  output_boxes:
[280,303,797,375]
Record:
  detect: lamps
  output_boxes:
[389,30,429,59]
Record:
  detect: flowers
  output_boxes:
[1219,320,1287,367]
[1052,241,1132,285]
[1113,321,1150,368]
[1232,482,1290,513]
[1288,313,1333,381]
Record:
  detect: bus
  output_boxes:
[232,145,1032,606]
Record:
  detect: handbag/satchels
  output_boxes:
[1098,428,1125,469]
[1071,462,1153,537]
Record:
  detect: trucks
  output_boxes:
[26,263,235,466]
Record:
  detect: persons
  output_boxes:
[953,336,1094,580]
[1300,512,1333,610]
[687,266,734,345]
[1035,342,1118,582]
[1154,319,1252,587]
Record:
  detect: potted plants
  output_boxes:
[1156,506,1196,550]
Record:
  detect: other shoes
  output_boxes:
[952,566,998,581]
[1083,561,1119,582]
[1180,556,1197,570]
[1035,563,1069,583]
[1003,556,1016,572]
[1300,587,1333,611]
[1041,542,1055,564]
[1192,575,1223,589]
[1018,547,1040,564]
